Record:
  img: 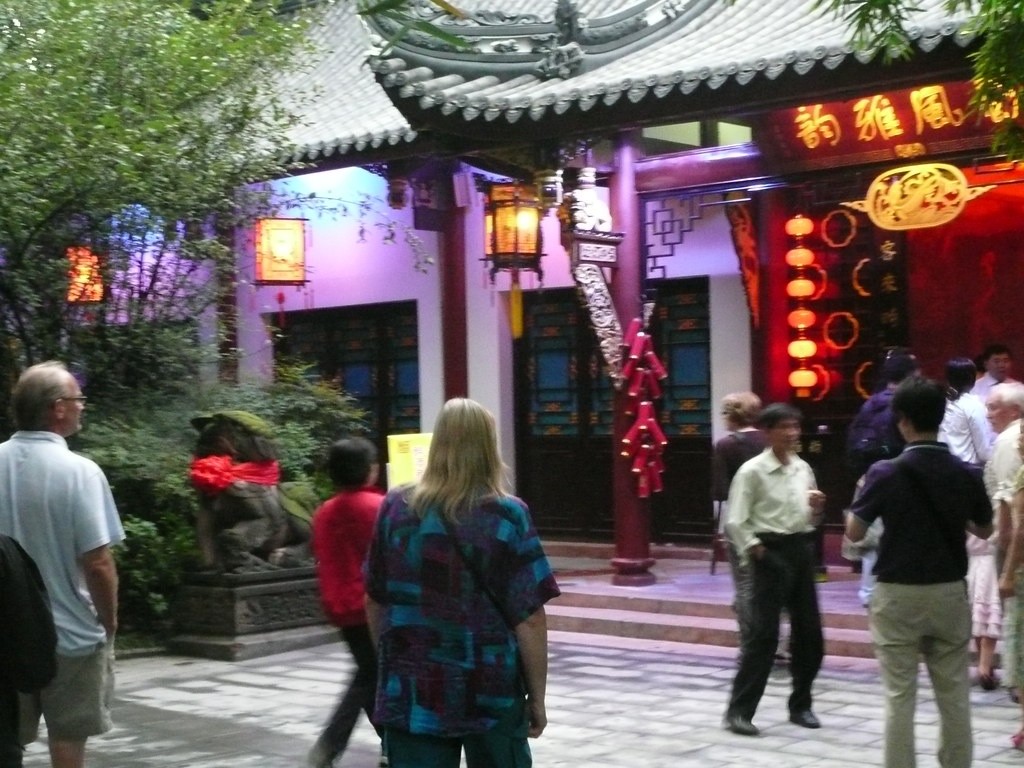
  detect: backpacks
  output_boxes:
[847,396,901,485]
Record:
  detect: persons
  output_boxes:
[297,434,394,768]
[996,409,1024,751]
[840,331,1016,611]
[360,394,561,768]
[719,401,828,738]
[0,358,127,768]
[709,388,793,668]
[846,371,995,768]
[0,528,61,768]
[981,377,1024,707]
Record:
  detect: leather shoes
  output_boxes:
[787,707,821,728]
[724,714,760,738]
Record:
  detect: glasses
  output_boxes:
[45,395,88,408]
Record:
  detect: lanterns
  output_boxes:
[785,277,817,299]
[788,366,819,399]
[787,335,818,362]
[477,175,552,287]
[65,240,109,306]
[784,245,816,270]
[787,307,818,330]
[252,213,312,289]
[783,213,815,238]
